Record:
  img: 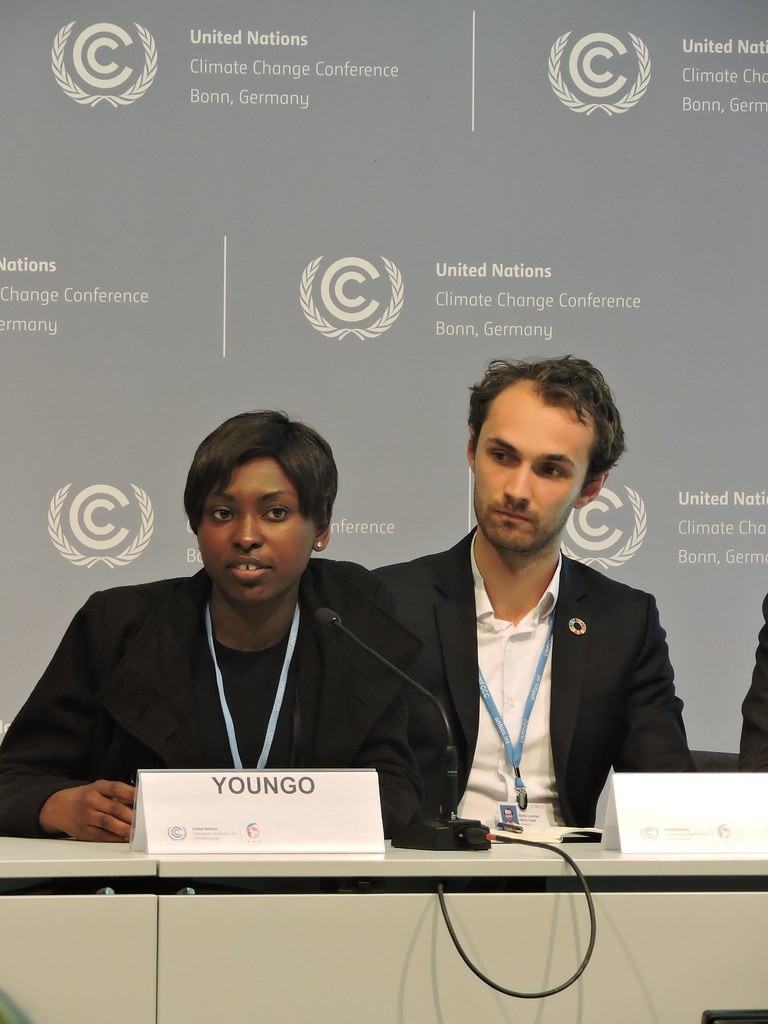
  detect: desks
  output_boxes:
[0,831,157,1024]
[149,834,766,1024]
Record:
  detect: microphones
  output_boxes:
[314,607,493,851]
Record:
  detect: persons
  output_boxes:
[503,808,518,824]
[370,354,698,835]
[0,409,426,842]
